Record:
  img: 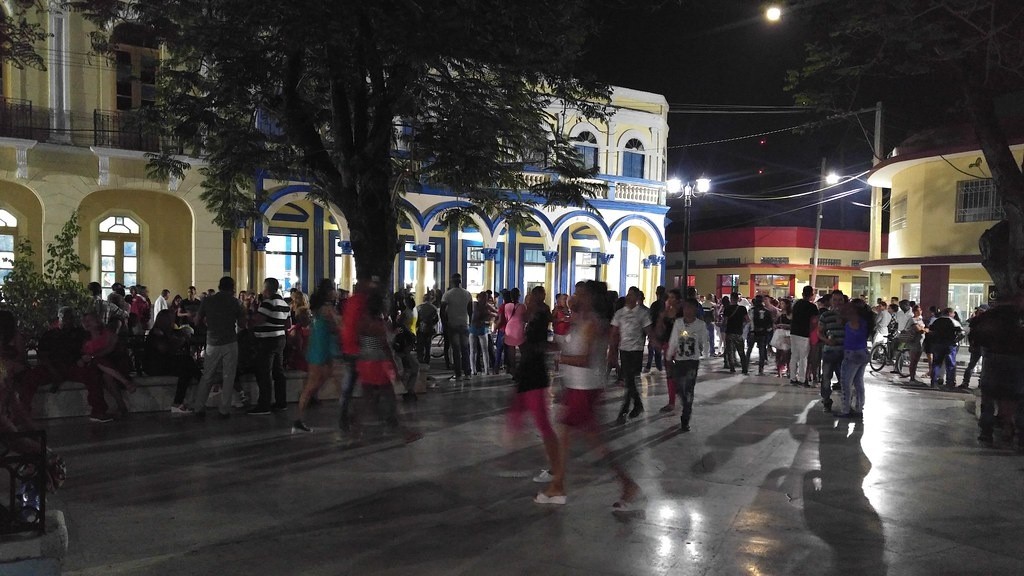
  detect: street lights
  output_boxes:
[668,172,712,302]
[810,157,840,293]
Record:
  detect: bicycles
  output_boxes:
[431,334,444,358]
[870,336,911,377]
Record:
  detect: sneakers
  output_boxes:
[533,469,553,482]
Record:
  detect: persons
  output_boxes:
[789,285,820,388]
[0,273,1024,513]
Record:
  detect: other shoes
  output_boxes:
[834,412,863,419]
[240,390,248,403]
[272,403,288,411]
[617,407,629,424]
[90,412,114,423]
[291,419,313,434]
[126,381,136,393]
[16,481,41,528]
[629,403,644,418]
[246,406,271,416]
[909,380,923,386]
[822,398,833,412]
[659,405,675,413]
[681,416,691,431]
[111,408,129,417]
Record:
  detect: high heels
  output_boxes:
[533,492,567,504]
[611,496,647,512]
[209,384,222,398]
[171,404,192,414]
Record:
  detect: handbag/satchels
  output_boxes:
[899,318,916,342]
[769,329,791,351]
